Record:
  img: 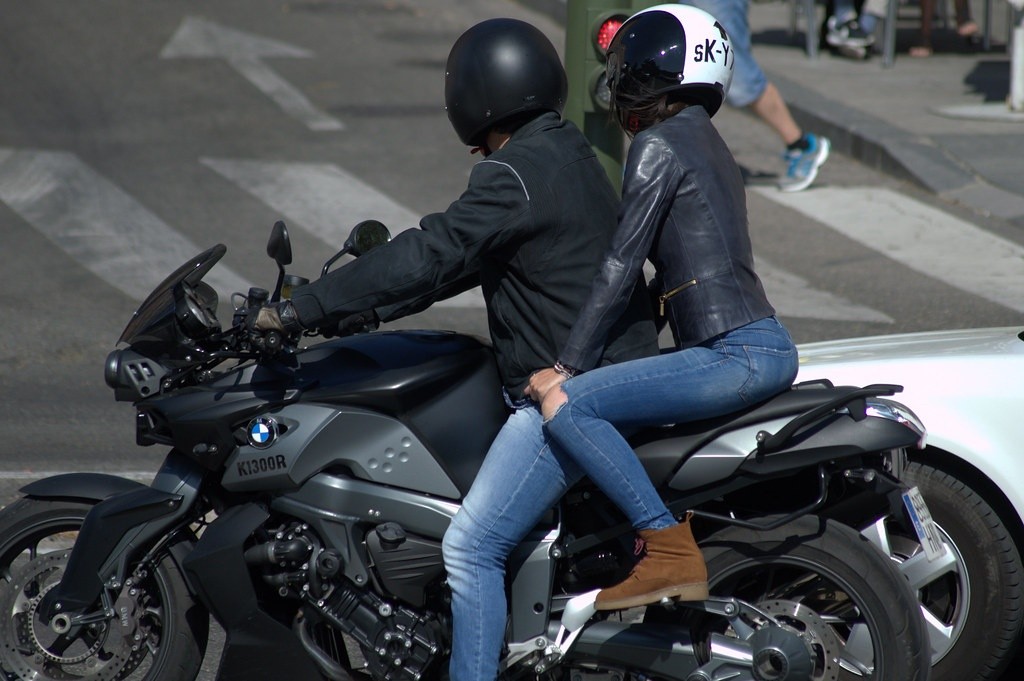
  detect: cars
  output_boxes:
[790,327,1024,681]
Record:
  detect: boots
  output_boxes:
[594,510,710,610]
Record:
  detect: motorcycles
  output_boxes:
[0,219,933,681]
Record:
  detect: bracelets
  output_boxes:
[553,360,575,379]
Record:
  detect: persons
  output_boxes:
[648,2,830,196]
[245,17,659,681]
[520,5,798,614]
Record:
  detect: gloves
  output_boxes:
[256,300,306,333]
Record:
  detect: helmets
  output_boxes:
[445,18,569,145]
[606,4,735,118]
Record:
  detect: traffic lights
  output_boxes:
[583,9,632,116]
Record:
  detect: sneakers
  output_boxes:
[779,132,832,192]
[824,17,877,60]
